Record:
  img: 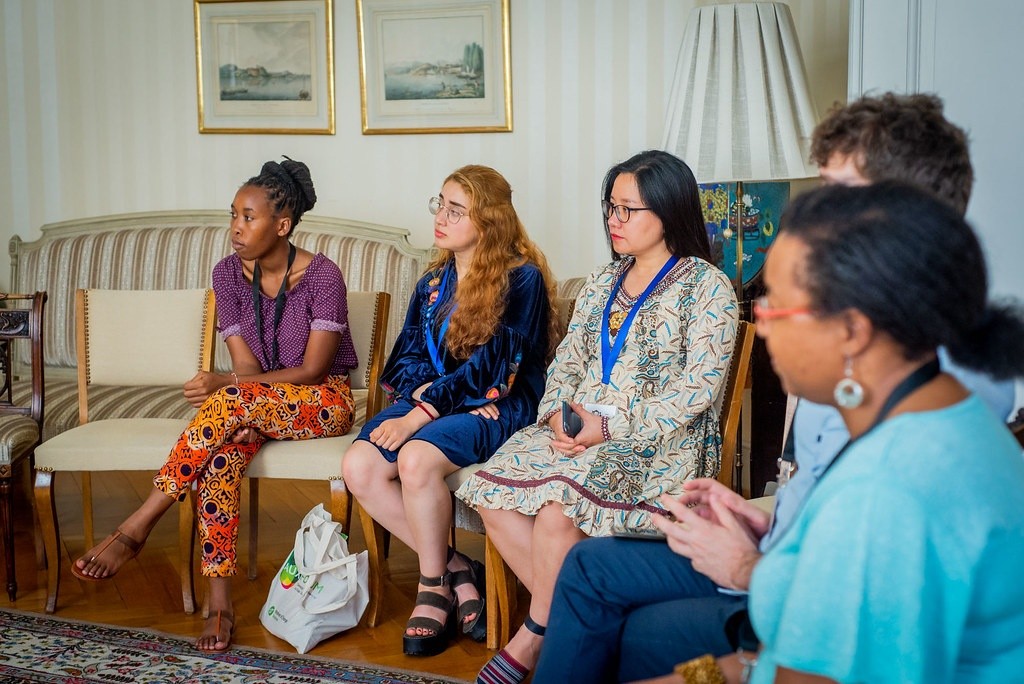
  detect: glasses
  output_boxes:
[428,197,470,224]
[754,293,822,328]
[601,200,652,223]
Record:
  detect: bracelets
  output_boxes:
[674,653,726,684]
[416,402,435,421]
[600,416,612,442]
[231,372,238,384]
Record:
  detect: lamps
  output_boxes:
[659,1,826,306]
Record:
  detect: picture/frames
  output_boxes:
[192,2,334,135]
[357,2,512,135]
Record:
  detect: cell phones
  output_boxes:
[562,402,582,438]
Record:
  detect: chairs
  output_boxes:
[354,277,586,651]
[243,291,393,581]
[490,319,757,658]
[32,287,215,615]
[0,292,48,600]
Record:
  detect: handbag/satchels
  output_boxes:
[258,502,370,654]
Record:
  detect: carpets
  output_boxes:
[1,613,467,684]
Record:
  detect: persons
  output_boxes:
[535,89,1015,684]
[342,164,553,656]
[633,181,1023,684]
[70,158,358,653]
[457,149,739,684]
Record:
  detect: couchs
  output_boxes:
[9,208,438,428]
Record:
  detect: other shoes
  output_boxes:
[474,610,547,684]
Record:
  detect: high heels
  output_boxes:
[403,544,486,657]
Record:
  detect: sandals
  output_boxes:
[196,610,237,655]
[71,528,147,582]
